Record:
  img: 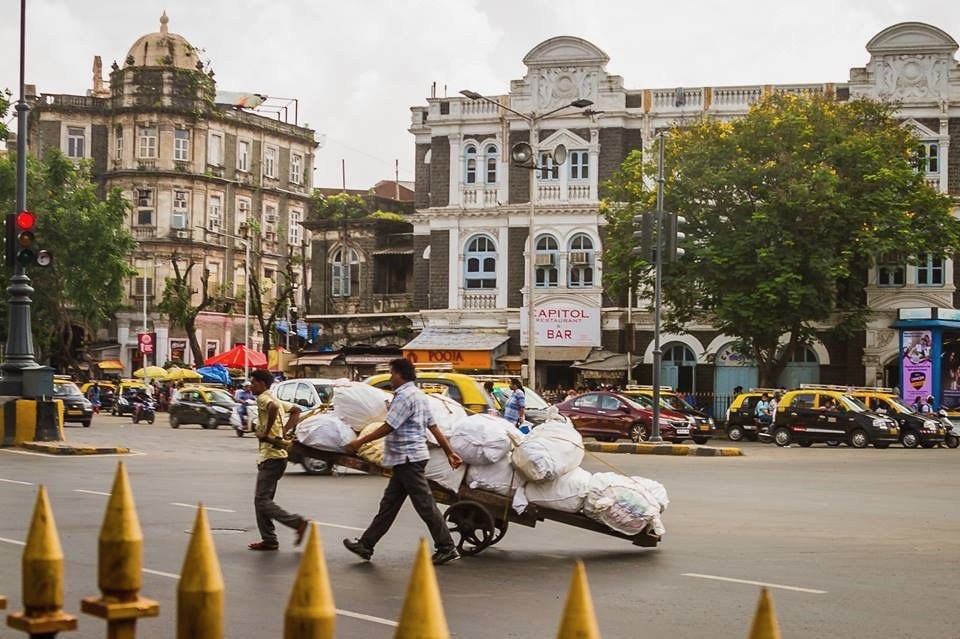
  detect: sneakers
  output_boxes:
[432,545,461,566]
[343,537,374,560]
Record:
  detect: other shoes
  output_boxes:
[247,542,278,550]
[296,520,308,545]
[938,445,949,448]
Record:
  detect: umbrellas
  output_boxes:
[132,342,287,390]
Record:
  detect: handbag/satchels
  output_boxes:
[516,417,529,434]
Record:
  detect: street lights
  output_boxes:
[197,224,252,388]
[458,89,594,390]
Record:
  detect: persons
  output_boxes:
[677,385,782,422]
[903,333,959,391]
[536,382,626,408]
[821,394,945,448]
[483,380,503,417]
[501,379,526,427]
[248,368,310,550]
[87,378,257,428]
[344,359,464,566]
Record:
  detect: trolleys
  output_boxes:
[259,377,670,554]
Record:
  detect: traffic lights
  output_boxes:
[665,212,689,263]
[14,211,38,268]
[632,212,655,263]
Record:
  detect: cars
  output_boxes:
[441,375,575,429]
[555,392,693,444]
[621,385,717,445]
[112,380,153,415]
[724,388,792,441]
[282,363,499,476]
[815,388,945,449]
[170,386,243,429]
[773,388,900,449]
[53,374,93,428]
[81,380,118,413]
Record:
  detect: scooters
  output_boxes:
[230,400,261,437]
[932,408,960,450]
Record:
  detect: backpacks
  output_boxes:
[918,404,930,414]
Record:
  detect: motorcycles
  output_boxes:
[753,416,775,443]
[132,400,157,425]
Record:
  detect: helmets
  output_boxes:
[927,394,935,404]
